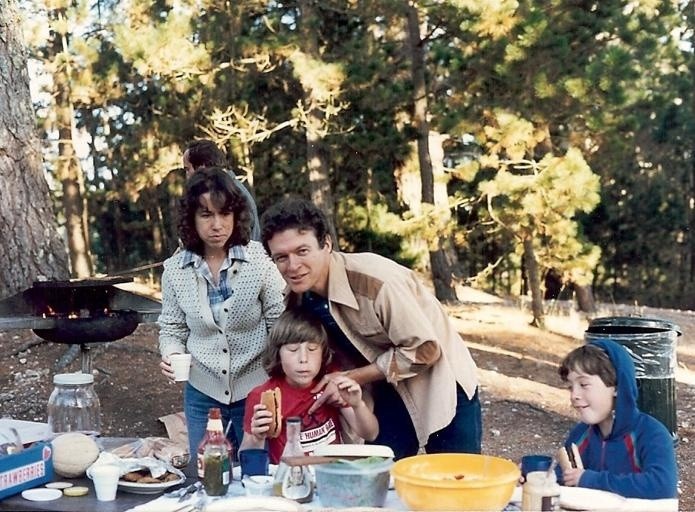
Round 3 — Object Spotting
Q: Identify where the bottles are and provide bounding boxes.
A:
[274,415,315,501]
[48,373,102,479]
[196,408,234,496]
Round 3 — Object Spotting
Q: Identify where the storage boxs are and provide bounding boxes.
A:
[1,440,56,500]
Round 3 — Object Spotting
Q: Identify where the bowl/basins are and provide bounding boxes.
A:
[388,452,521,509]
[309,454,390,507]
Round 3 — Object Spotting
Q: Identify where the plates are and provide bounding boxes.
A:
[87,457,186,494]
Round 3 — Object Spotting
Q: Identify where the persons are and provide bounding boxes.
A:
[516,338,678,500]
[155,167,288,468]
[183,139,261,467]
[259,199,484,463]
[237,307,380,465]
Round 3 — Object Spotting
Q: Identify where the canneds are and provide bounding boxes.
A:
[203,445,230,496]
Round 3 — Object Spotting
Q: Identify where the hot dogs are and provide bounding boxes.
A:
[558,442,584,472]
[260,386,282,438]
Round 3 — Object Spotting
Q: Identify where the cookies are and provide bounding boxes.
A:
[122,471,180,483]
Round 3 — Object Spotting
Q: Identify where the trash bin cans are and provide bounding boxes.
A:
[584,316,683,448]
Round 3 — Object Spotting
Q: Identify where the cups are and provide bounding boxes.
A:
[241,474,276,496]
[167,352,194,384]
[88,464,120,502]
[237,445,270,477]
